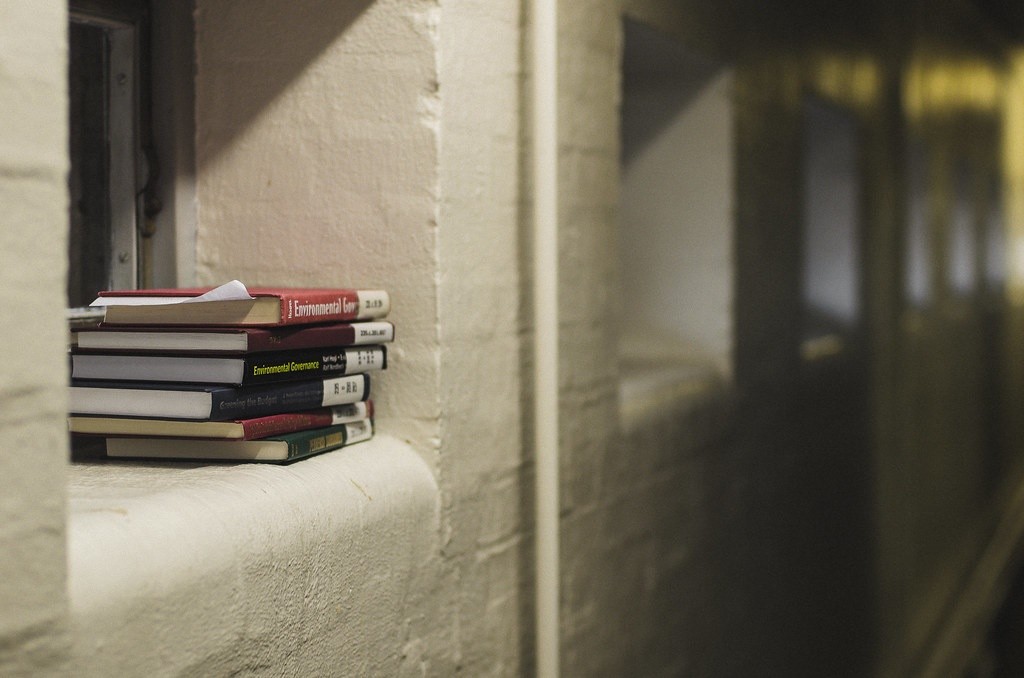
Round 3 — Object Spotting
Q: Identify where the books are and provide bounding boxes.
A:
[65,279,395,465]
